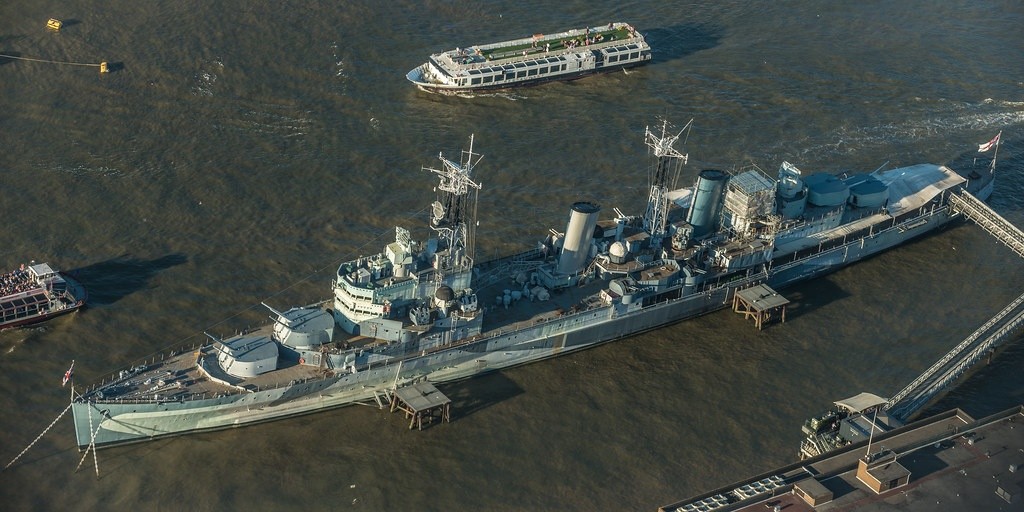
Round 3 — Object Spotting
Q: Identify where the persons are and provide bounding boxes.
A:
[532,32,601,52]
[456,46,482,64]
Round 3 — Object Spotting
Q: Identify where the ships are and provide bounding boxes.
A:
[70,113,997,454]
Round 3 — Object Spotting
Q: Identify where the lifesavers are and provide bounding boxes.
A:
[298,358,305,364]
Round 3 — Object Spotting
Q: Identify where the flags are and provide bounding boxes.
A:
[62,365,74,386]
[978,133,1000,153]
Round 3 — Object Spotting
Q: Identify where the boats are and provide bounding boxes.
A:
[406,22,652,92]
[0,261,89,330]
